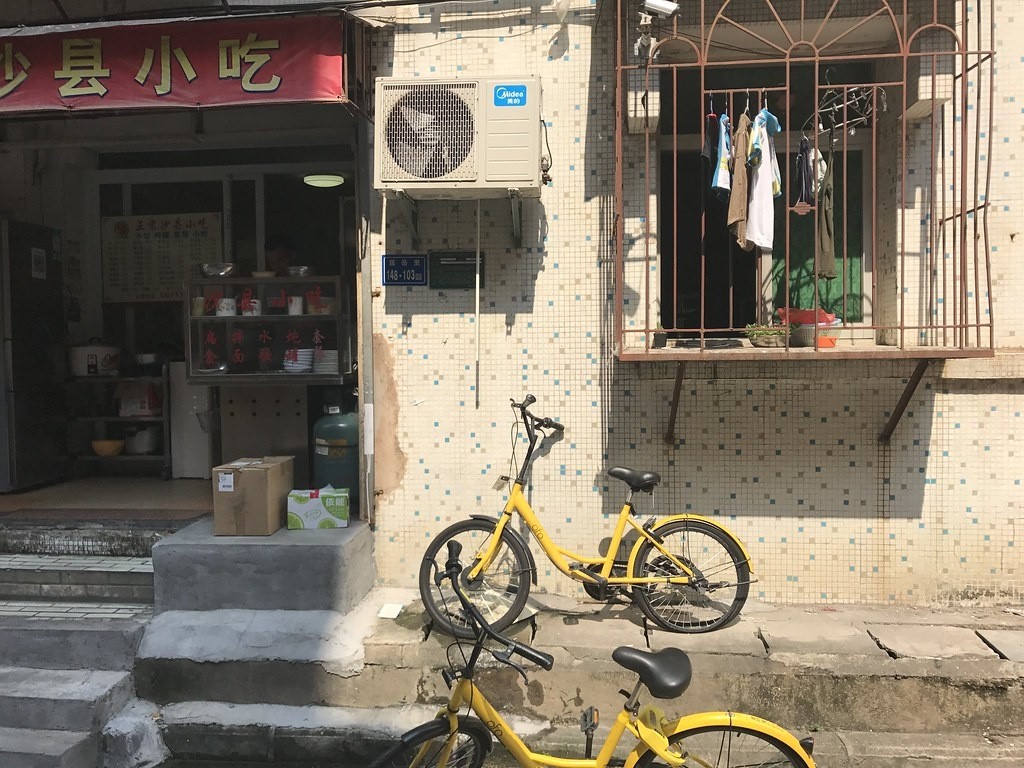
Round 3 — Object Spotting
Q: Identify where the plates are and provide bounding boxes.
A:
[200,369,229,374]
[283,349,337,372]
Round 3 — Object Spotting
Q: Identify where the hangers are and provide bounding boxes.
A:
[797,68,886,139]
[708,87,769,120]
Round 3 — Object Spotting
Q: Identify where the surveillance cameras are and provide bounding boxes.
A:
[643,0,680,18]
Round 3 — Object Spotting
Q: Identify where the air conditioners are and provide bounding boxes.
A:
[368,69,550,204]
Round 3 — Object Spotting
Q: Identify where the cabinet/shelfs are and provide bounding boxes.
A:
[62,359,172,484]
[179,265,349,393]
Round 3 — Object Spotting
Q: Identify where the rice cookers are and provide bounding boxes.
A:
[71,337,122,378]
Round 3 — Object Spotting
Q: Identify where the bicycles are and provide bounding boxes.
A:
[419,394,759,640]
[364,539,816,768]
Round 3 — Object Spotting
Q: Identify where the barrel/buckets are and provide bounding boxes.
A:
[125,423,161,455]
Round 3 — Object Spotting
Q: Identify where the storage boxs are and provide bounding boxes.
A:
[195,449,374,542]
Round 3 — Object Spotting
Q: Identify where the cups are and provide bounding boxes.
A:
[242,300,262,316]
[190,297,204,316]
[288,296,303,315]
[215,298,237,316]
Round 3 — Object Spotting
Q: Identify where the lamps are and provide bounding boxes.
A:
[297,170,349,191]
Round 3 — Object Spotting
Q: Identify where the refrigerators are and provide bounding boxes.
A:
[0,219,75,495]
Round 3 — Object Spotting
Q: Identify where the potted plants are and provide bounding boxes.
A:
[740,319,801,348]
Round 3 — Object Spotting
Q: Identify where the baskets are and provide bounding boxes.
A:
[791,318,842,347]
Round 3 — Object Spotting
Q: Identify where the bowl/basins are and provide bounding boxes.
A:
[306,296,336,315]
[92,440,125,456]
[288,265,312,277]
[251,271,277,277]
[199,263,237,279]
